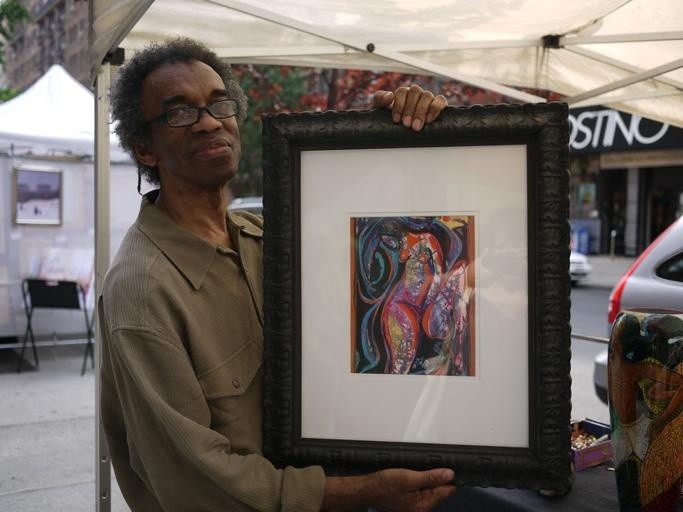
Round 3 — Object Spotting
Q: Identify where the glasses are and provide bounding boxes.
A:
[141,97,240,129]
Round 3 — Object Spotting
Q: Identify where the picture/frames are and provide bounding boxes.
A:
[260,101,572,491]
[10,164,62,227]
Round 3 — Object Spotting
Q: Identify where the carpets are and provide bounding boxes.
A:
[0,348,38,373]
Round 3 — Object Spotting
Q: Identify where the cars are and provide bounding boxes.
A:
[569,249,591,286]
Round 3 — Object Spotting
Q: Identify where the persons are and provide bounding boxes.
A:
[96,36,458,512]
[607,314,682,512]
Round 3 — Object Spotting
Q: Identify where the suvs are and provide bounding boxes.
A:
[594,213,683,406]
